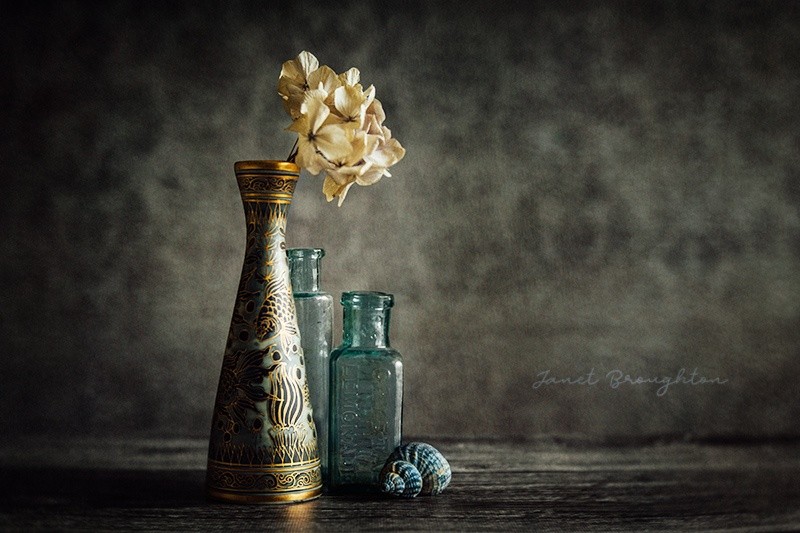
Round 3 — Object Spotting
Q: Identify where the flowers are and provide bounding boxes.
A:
[276,49,406,206]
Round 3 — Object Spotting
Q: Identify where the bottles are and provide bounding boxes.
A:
[286,247,334,482]
[329,290,404,494]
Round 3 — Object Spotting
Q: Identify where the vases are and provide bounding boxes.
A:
[206,160,323,506]
